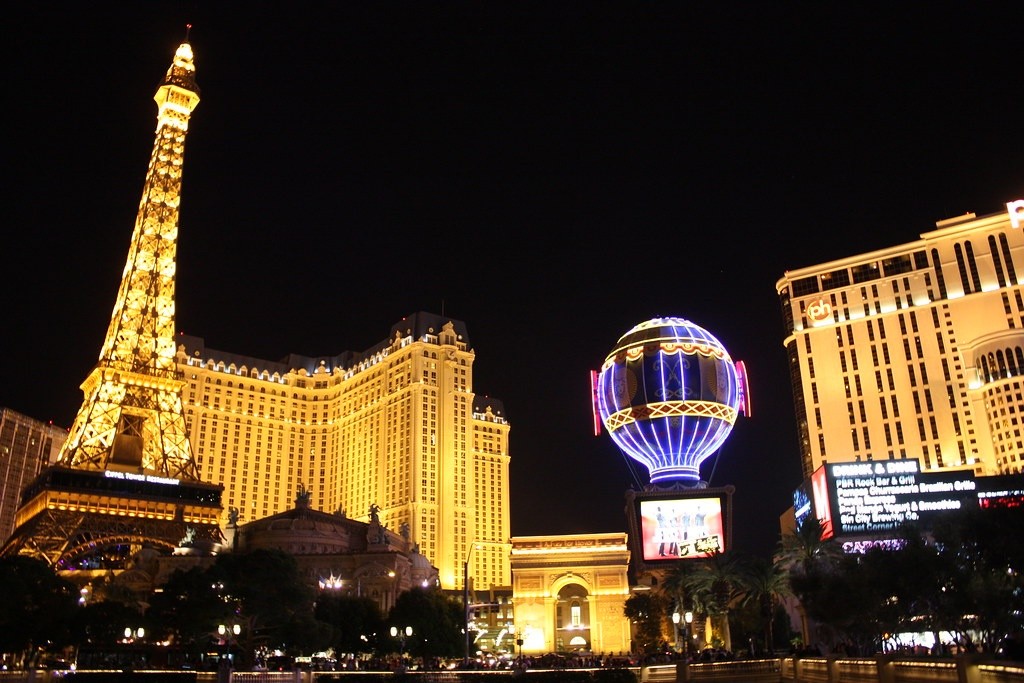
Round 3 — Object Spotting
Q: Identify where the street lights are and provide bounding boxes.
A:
[672,612,694,661]
[124,627,146,666]
[390,626,414,668]
[218,624,241,668]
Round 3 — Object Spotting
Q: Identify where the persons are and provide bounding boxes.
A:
[500,641,732,670]
[845,639,1015,665]
[653,504,709,556]
